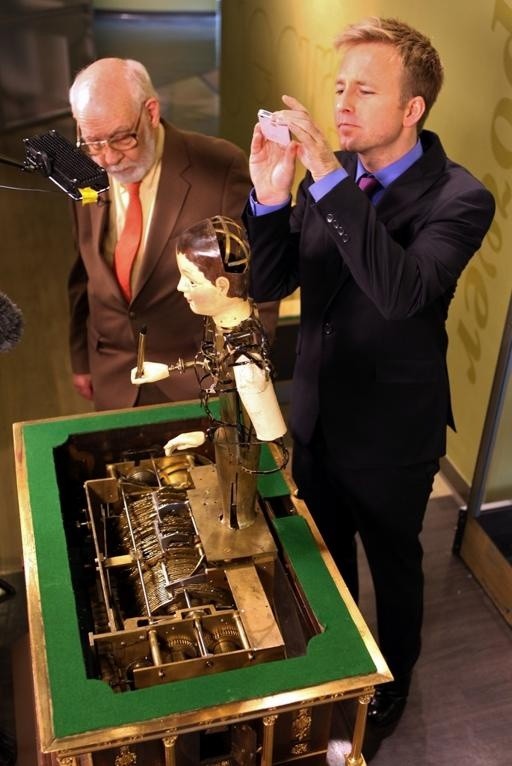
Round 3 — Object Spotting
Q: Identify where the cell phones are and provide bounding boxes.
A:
[257,109,292,146]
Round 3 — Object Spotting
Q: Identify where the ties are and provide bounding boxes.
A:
[358,177,376,193]
[114,181,142,303]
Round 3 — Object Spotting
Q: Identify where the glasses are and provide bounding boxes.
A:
[76,106,144,156]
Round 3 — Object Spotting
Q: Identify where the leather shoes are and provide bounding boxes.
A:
[367,690,405,736]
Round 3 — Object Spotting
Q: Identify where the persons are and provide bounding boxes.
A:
[66,56,280,411]
[242,18,496,728]
[127,217,287,443]
